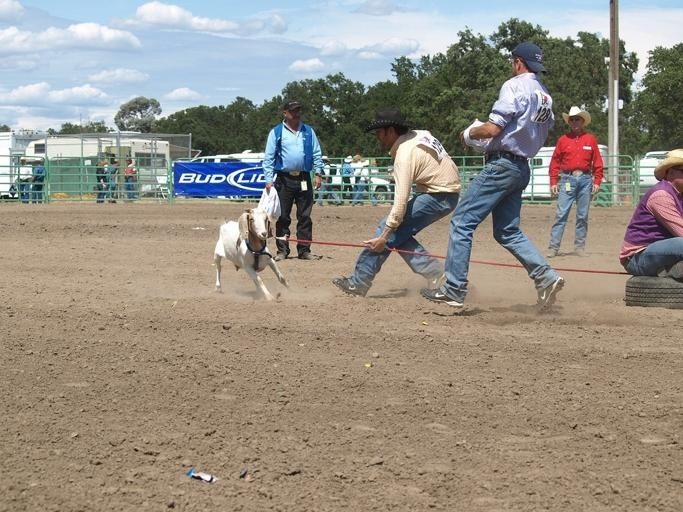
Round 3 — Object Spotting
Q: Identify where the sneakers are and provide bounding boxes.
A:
[300,252,317,260]
[575,249,587,257]
[547,250,561,258]
[657,260,683,280]
[275,253,287,260]
[537,277,564,312]
[428,273,445,289]
[332,277,368,298]
[420,289,463,307]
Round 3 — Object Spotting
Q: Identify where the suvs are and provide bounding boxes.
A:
[631,148,674,187]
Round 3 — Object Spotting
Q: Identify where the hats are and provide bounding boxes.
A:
[284,102,302,111]
[512,42,548,73]
[343,155,369,166]
[321,155,329,161]
[363,108,413,134]
[654,148,683,181]
[562,105,591,128]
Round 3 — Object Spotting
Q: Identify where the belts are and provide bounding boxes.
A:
[283,170,304,176]
[623,257,632,269]
[491,151,527,160]
[563,169,587,176]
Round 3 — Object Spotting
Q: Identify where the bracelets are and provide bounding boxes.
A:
[315,173,321,177]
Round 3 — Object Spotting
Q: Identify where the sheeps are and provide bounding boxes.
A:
[212,207,292,302]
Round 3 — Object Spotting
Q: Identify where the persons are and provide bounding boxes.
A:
[93,149,138,205]
[31,158,47,204]
[315,154,342,207]
[261,102,327,261]
[333,107,463,298]
[418,42,564,315]
[17,158,32,203]
[617,148,682,279]
[546,106,603,258]
[339,154,395,207]
